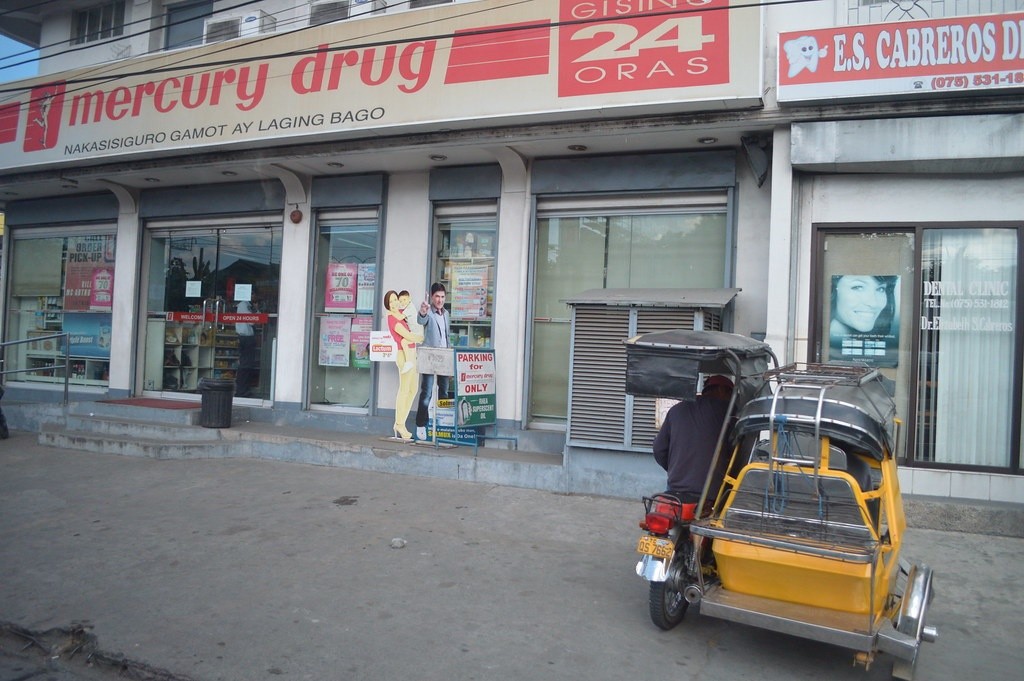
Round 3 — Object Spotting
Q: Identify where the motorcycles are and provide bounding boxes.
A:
[620,330,939,680]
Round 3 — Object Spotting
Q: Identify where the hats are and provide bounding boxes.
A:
[701,374,735,394]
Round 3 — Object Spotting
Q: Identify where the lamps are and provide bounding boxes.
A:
[740,135,773,188]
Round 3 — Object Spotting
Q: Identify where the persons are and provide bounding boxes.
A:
[384,290,423,439]
[387,290,424,374]
[235,288,258,398]
[416,283,451,439]
[831,275,896,338]
[653,376,743,573]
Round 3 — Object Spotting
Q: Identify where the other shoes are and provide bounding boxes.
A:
[402,363,413,373]
[417,426,426,440]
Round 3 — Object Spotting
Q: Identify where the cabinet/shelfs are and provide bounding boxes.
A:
[437,223,497,392]
[17,250,112,386]
[144,317,262,391]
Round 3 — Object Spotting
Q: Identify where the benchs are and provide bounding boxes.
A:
[745,444,877,534]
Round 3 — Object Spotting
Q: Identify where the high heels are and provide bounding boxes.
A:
[393,423,412,440]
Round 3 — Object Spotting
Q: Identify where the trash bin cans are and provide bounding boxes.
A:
[200,377,235,429]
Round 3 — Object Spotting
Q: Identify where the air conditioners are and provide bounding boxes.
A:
[202,8,276,45]
[308,0,388,27]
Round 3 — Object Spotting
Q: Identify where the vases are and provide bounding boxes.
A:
[200,377,234,428]
